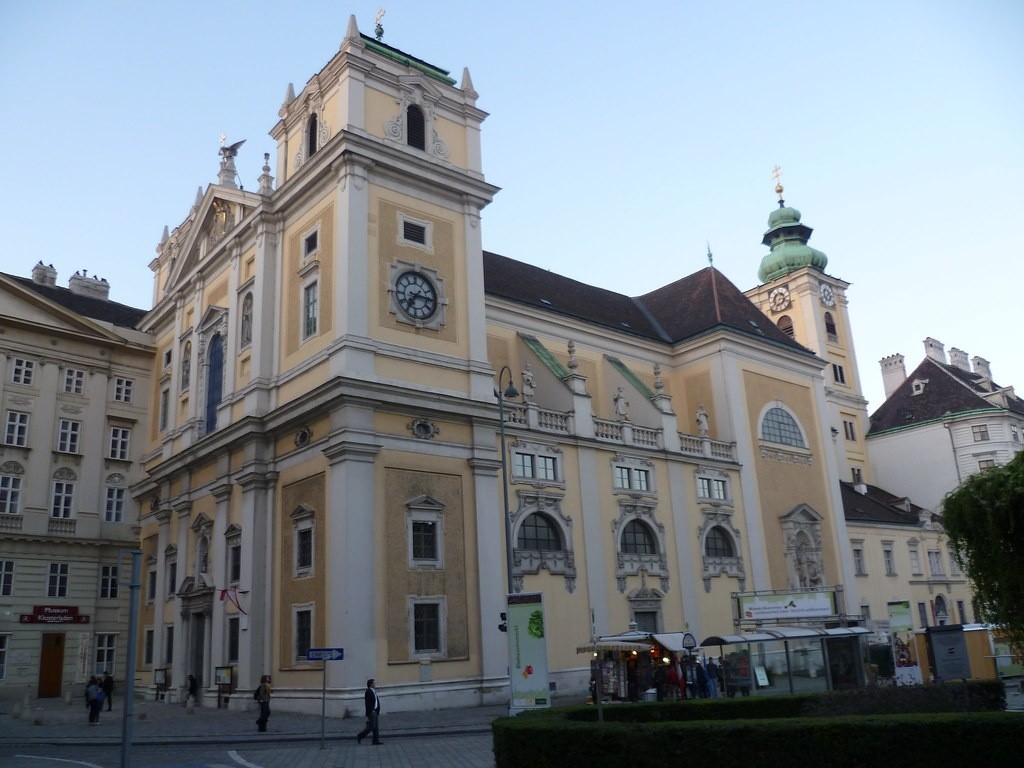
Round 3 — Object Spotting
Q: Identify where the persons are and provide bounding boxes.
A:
[357,678,385,745]
[614,387,631,421]
[182,675,198,707]
[255,675,272,732]
[84,670,114,725]
[521,363,535,404]
[695,404,709,436]
[589,651,751,704]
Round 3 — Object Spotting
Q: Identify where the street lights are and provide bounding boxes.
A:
[498,366,519,595]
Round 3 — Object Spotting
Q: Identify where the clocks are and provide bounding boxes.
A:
[820,284,835,307]
[392,269,444,324]
[769,287,791,313]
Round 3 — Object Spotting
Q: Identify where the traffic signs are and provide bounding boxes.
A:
[308,648,344,748]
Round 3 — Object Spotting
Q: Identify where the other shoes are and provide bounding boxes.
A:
[373,741,384,745]
[357,734,361,745]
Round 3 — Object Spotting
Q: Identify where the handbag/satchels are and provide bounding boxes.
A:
[95,686,104,702]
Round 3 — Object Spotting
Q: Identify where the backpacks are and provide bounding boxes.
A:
[254,685,261,700]
